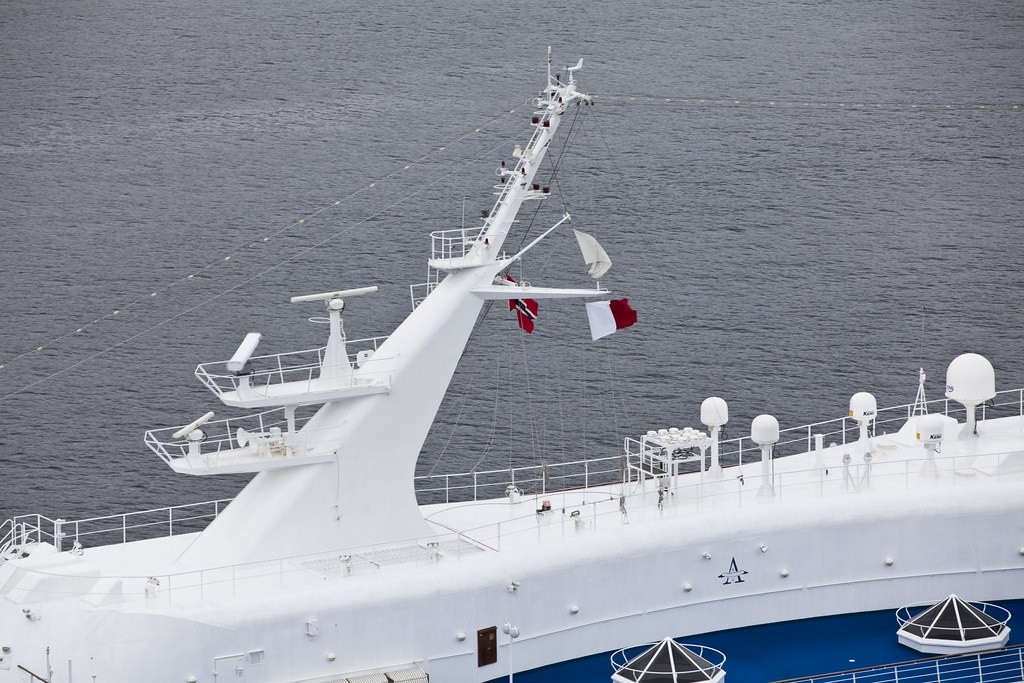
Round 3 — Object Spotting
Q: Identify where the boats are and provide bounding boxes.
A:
[0,46,1024,683]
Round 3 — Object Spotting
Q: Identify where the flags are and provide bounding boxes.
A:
[586,298,637,340]
[573,229,612,279]
[507,275,538,334]
